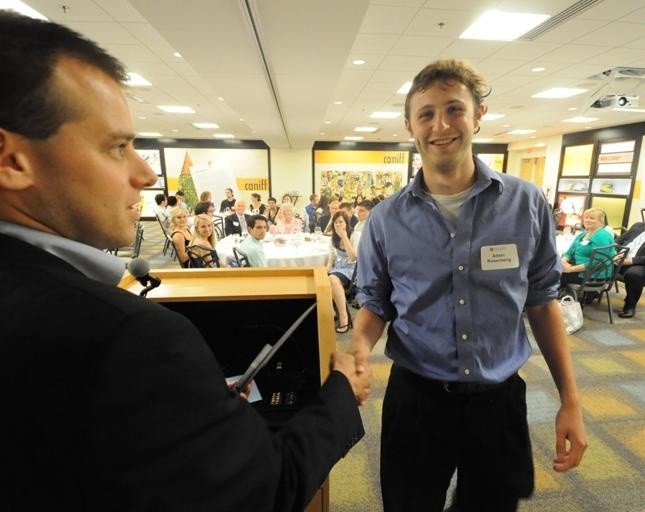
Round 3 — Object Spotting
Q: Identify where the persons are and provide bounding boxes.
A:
[560,209,613,286]
[339,203,357,237]
[190,202,214,235]
[153,191,184,212]
[200,190,212,202]
[0,9,371,512]
[321,200,340,236]
[269,203,302,235]
[250,193,266,215]
[339,195,384,204]
[349,200,373,242]
[236,215,268,267]
[188,214,216,267]
[326,210,357,333]
[219,188,236,212]
[348,57,589,512]
[224,199,251,236]
[267,197,280,225]
[282,194,292,203]
[170,206,192,268]
[305,194,326,234]
[614,222,645,318]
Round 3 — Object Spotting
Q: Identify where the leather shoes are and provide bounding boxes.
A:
[618,308,635,318]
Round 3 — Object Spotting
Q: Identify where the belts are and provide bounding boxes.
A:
[415,381,509,394]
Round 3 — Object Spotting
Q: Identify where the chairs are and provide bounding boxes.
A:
[232,247,250,267]
[111,231,144,259]
[185,245,220,268]
[613,227,628,241]
[166,234,187,269]
[561,244,630,324]
[213,214,223,239]
[155,213,169,256]
[344,262,358,329]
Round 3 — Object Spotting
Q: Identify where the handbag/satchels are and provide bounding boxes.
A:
[558,294,584,335]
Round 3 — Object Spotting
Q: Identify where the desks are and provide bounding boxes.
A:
[117,265,335,512]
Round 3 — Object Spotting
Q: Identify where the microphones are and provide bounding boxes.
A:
[128,258,161,298]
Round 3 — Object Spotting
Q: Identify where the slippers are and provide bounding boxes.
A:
[336,319,349,334]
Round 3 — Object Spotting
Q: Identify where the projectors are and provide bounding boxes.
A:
[600,92,640,111]
